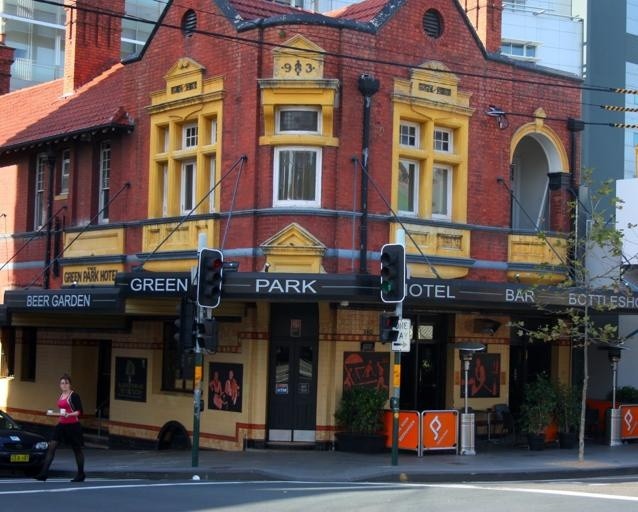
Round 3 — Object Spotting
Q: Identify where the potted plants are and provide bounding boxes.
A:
[333,382,389,453]
[518,369,583,450]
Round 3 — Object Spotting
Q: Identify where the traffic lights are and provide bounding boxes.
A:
[198,317,219,354]
[377,240,406,304]
[376,311,400,346]
[194,246,225,309]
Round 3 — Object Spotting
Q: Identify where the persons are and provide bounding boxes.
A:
[210,369,239,410]
[363,359,388,391]
[34,373,85,482]
[472,357,487,396]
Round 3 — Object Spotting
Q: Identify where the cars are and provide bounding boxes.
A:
[0,409,50,479]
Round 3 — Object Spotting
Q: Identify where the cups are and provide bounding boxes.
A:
[60,409,66,415]
[47,409,53,414]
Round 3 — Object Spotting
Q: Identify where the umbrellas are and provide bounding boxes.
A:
[345,353,363,364]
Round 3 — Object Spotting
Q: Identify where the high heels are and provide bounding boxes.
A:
[70,475,86,481]
[33,474,47,481]
